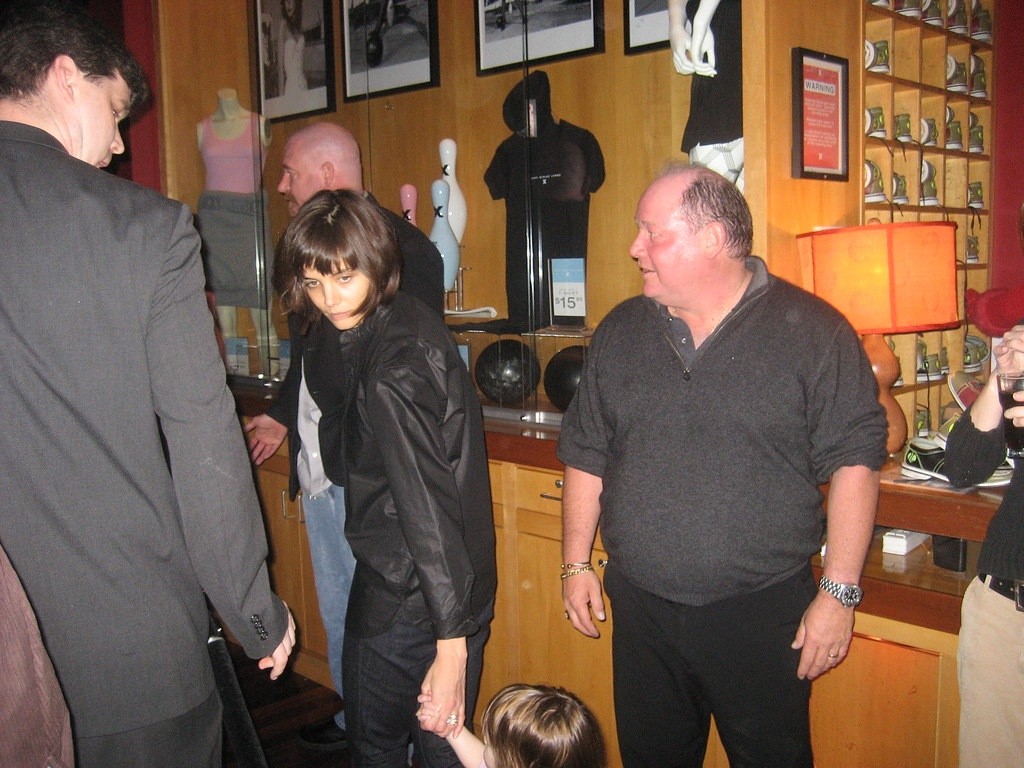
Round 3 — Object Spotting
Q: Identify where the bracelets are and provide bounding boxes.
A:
[561,556,593,579]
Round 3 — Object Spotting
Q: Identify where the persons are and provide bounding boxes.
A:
[196,88,281,377]
[667,0,743,194]
[559,162,888,767]
[0,0,297,768]
[944,318,1024,768]
[277,0,308,96]
[276,191,496,768]
[243,122,445,768]
[417,683,606,768]
[0,544,75,768]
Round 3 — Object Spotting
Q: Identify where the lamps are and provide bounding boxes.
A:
[795,221,960,453]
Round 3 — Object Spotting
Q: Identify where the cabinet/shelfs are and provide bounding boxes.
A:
[848,0,997,448]
[210,413,942,768]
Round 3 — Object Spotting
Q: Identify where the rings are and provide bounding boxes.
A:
[447,714,459,725]
[565,613,570,620]
[828,654,838,658]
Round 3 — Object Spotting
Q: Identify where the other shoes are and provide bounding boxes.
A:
[295,717,348,750]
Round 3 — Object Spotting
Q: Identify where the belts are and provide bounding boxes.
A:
[977,571,1024,612]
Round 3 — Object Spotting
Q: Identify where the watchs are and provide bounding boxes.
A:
[819,575,864,606]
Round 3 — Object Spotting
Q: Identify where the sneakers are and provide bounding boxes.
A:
[948,371,987,410]
[883,335,987,388]
[901,401,1014,488]
[860,0,992,266]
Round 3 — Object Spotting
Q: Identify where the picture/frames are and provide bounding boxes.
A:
[473,0,607,77]
[253,0,337,124]
[622,0,692,57]
[339,0,440,104]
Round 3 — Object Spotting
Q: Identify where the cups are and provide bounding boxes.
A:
[997,372,1024,458]
